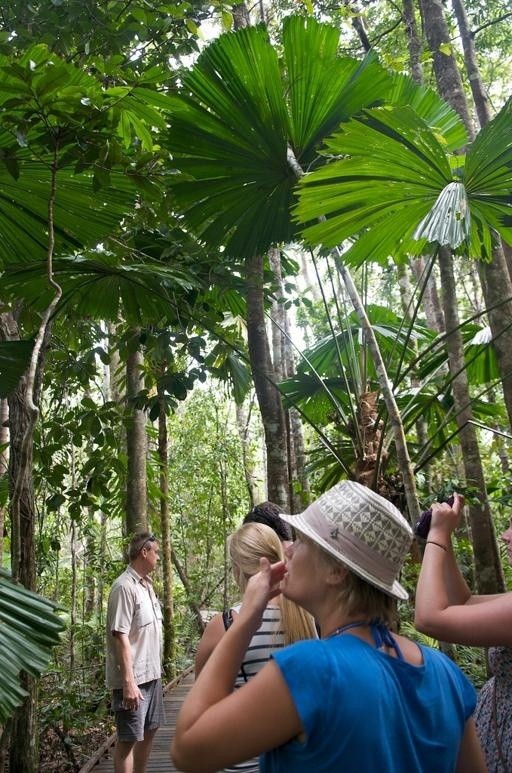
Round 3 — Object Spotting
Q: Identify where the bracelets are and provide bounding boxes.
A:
[425,541,447,551]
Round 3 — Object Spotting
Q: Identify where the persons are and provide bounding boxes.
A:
[171,480,487,773]
[243,502,293,553]
[414,492,512,773]
[195,522,319,773]
[107,532,168,772]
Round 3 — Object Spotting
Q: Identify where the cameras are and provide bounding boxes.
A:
[446,494,455,508]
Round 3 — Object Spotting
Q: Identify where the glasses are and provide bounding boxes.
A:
[251,506,273,523]
[139,535,155,550]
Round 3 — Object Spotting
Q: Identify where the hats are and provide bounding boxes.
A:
[278,477,416,603]
